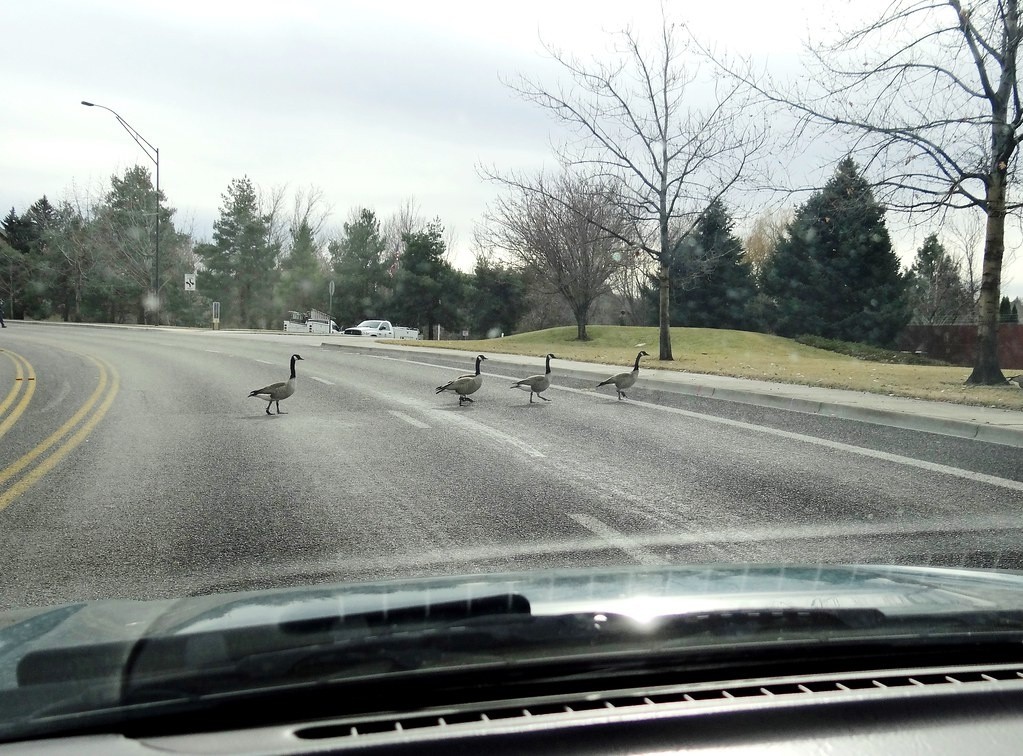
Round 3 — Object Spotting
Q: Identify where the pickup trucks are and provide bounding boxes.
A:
[344,320,419,341]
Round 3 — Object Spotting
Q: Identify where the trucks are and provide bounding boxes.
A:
[284,309,342,334]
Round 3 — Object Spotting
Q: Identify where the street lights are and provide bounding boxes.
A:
[81,101,159,328]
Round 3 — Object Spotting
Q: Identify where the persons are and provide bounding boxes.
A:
[0,299,7,328]
[619,310,626,326]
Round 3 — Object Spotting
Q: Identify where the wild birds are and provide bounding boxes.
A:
[247,353,305,416]
[509,353,557,404]
[435,355,489,406]
[595,350,651,400]
